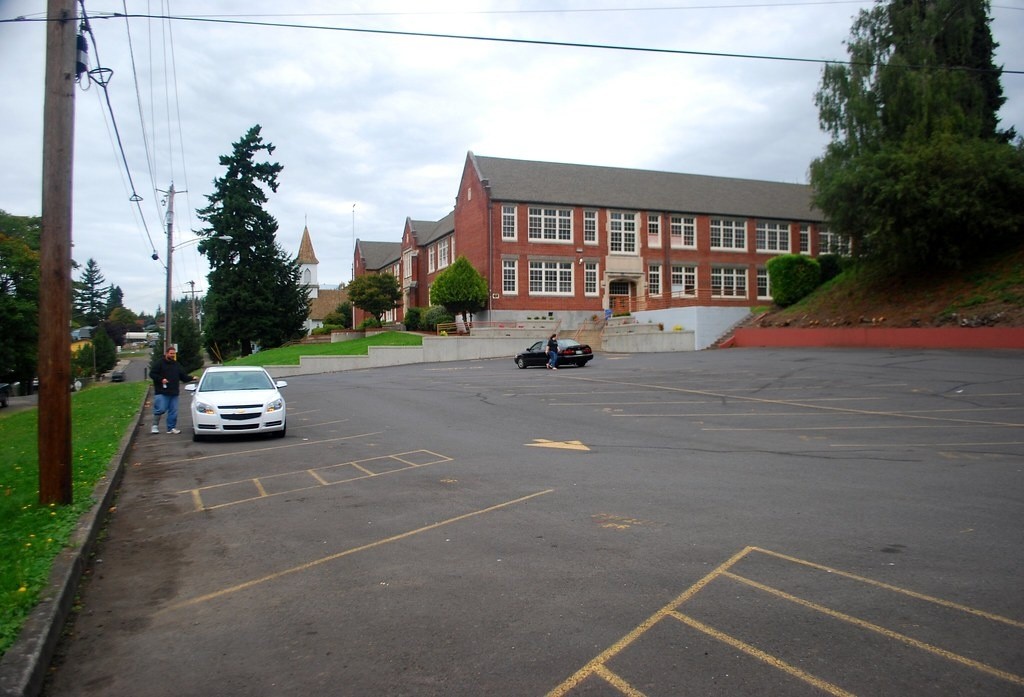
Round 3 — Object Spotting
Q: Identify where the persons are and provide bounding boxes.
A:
[545,335,560,370]
[603,308,612,320]
[148,347,201,435]
[439,328,448,337]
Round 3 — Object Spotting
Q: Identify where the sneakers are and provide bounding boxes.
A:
[167,428,182,434]
[150,424,159,433]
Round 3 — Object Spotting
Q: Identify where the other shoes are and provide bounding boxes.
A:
[546,363,558,370]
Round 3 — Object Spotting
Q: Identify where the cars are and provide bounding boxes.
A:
[512,338,593,369]
[0,382,11,409]
[184,365,288,440]
[149,341,155,347]
[33,378,40,388]
[113,371,126,381]
